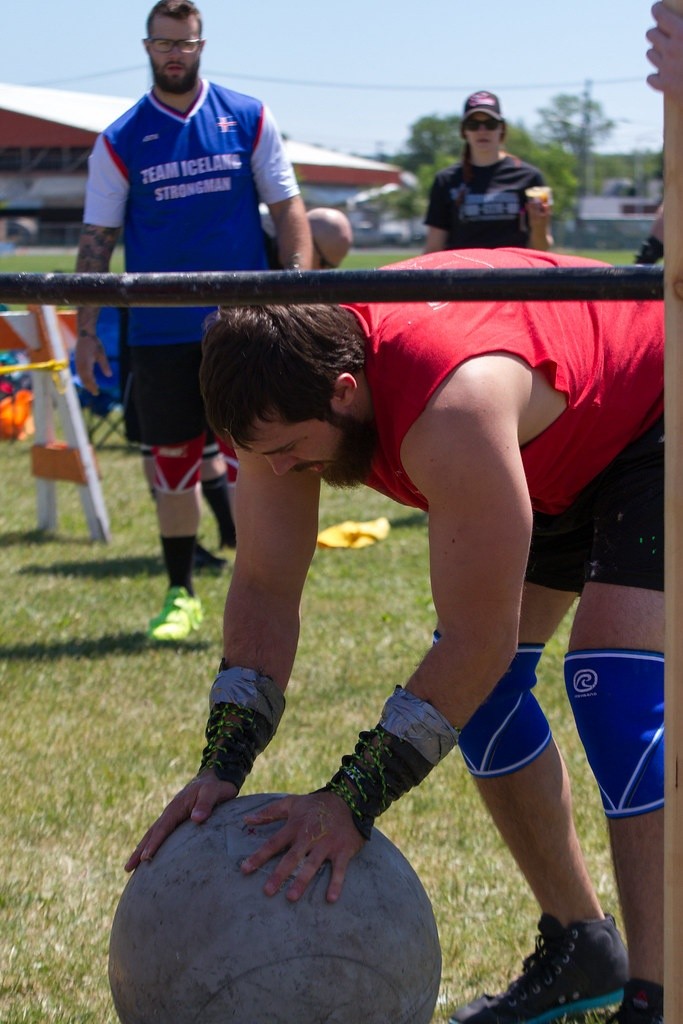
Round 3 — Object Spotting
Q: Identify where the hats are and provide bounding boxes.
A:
[464,91,503,122]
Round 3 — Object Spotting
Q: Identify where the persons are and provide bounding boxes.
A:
[140,201,354,572]
[76,0,315,641]
[634,1,682,265]
[422,90,554,257]
[123,247,666,1024]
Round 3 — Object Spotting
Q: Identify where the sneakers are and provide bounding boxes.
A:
[605,979,665,1024]
[447,912,628,1024]
[147,586,203,640]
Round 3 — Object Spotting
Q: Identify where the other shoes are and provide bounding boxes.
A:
[189,533,237,573]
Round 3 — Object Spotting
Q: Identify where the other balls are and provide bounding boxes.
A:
[109,792,442,1024]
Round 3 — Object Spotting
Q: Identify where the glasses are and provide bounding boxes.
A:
[148,38,201,54]
[466,118,504,131]
[313,240,337,270]
